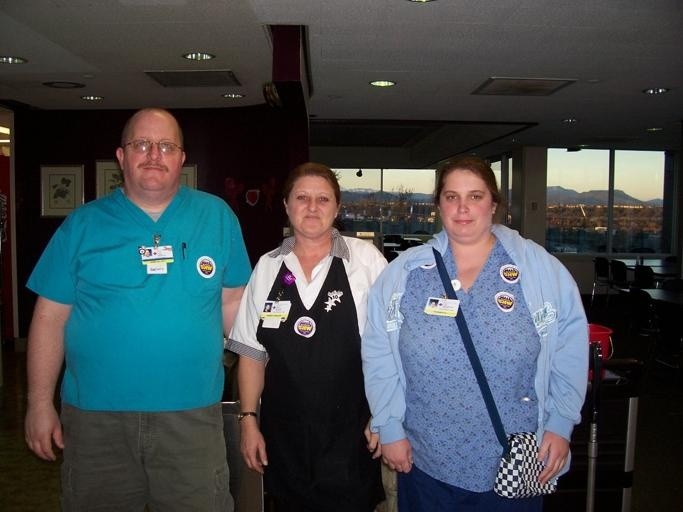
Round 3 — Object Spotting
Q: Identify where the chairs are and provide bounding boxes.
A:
[590,255,668,367]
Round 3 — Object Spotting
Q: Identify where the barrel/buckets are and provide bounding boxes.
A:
[587,323,615,379]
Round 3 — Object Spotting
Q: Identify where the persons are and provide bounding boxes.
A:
[359,154,592,511]
[22,106,255,511]
[226,161,391,512]
[143,249,152,259]
[263,303,270,312]
[429,299,438,309]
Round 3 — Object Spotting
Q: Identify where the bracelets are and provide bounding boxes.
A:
[236,411,259,423]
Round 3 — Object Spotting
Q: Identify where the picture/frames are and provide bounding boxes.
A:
[178,162,199,189]
[38,159,118,219]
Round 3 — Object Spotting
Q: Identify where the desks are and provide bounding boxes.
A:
[607,258,683,306]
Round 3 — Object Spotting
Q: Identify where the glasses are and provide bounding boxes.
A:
[121,137,183,155]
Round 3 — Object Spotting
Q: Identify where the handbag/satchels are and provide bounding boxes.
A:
[493,430,561,500]
